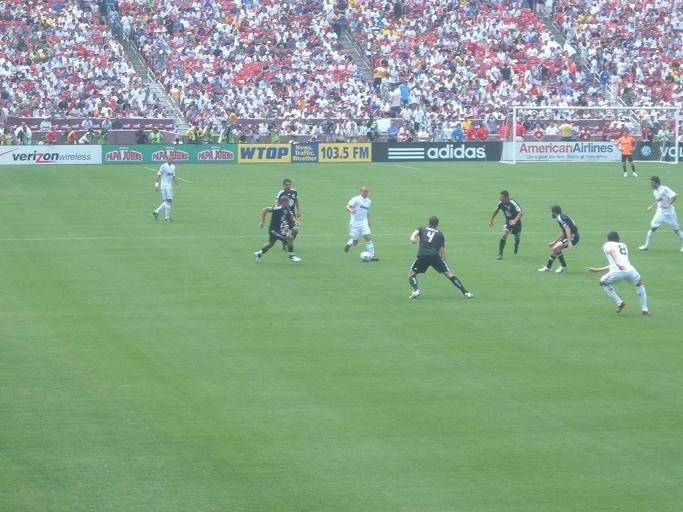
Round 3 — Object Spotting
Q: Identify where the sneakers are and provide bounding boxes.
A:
[345,243,350,251]
[255,250,262,262]
[538,265,567,273]
[289,255,300,262]
[409,289,420,299]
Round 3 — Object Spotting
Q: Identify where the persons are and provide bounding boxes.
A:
[538,205,580,273]
[409,216,474,299]
[153,155,178,221]
[254,196,302,262]
[616,131,639,178]
[276,179,300,250]
[586,231,650,315]
[488,190,523,259]
[637,176,683,252]
[346,187,379,261]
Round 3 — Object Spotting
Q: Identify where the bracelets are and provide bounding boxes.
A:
[155,182,159,187]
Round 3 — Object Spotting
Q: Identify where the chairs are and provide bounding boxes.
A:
[0,0,683,144]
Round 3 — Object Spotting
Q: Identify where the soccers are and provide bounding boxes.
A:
[361,252,371,262]
